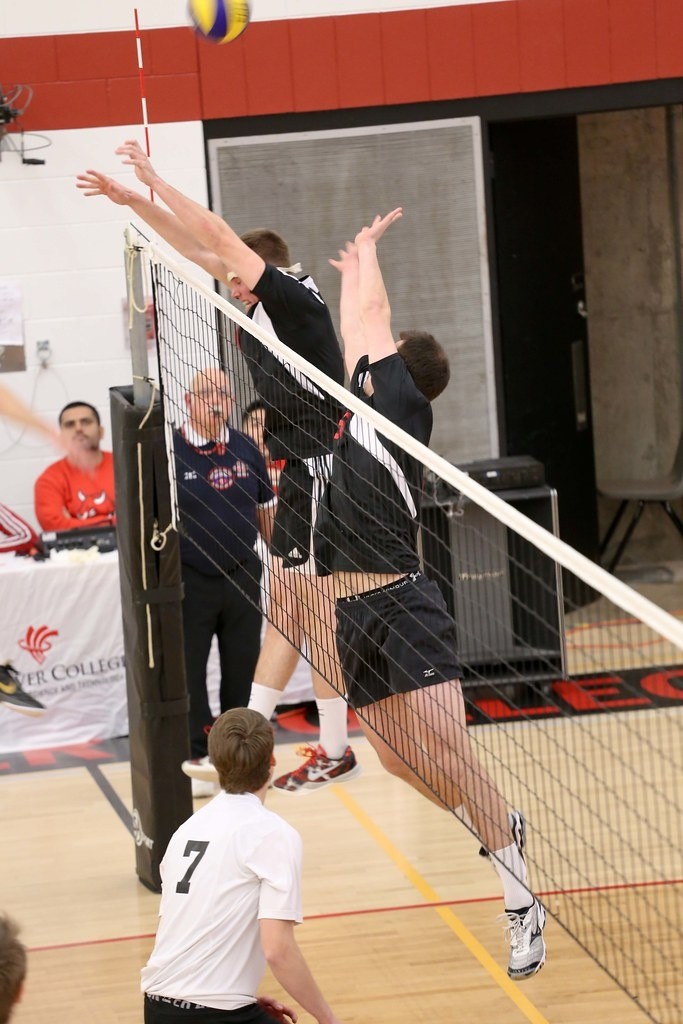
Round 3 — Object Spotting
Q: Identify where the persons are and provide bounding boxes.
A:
[240,399,287,496]
[313,207,548,983]
[34,401,117,533]
[168,365,278,795]
[75,138,362,797]
[0,500,38,555]
[140,705,338,1024]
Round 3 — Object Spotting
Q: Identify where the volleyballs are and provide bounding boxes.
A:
[183,0,252,43]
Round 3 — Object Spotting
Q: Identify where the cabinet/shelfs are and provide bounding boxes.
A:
[416,484,568,688]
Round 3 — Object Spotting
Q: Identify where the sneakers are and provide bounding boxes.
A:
[2,663,51,715]
[182,744,361,795]
[478,808,549,978]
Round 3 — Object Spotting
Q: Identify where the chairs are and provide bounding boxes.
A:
[592,424,683,575]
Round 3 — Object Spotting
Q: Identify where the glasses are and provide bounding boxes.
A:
[189,389,232,401]
